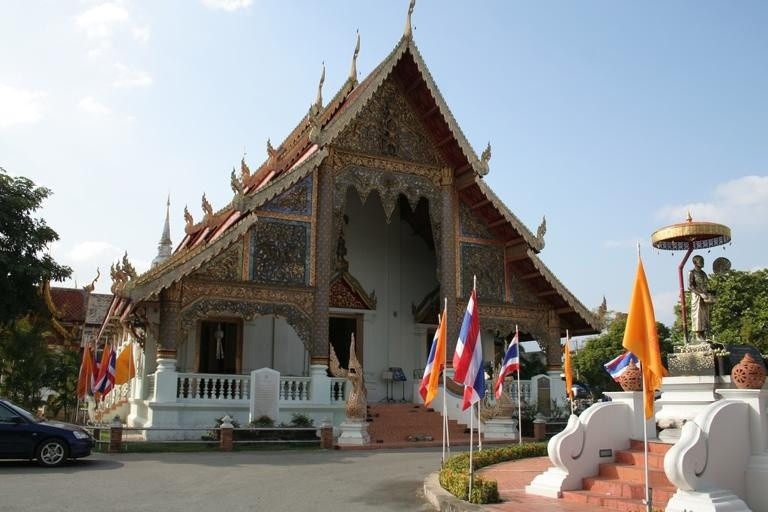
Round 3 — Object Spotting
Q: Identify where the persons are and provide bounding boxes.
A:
[687,255,715,343]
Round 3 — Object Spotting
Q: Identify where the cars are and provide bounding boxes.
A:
[0,396,96,467]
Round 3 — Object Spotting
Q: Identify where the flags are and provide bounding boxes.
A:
[564,341,572,399]
[494,331,521,400]
[621,254,663,419]
[602,350,640,384]
[418,287,486,413]
[76,340,135,402]
